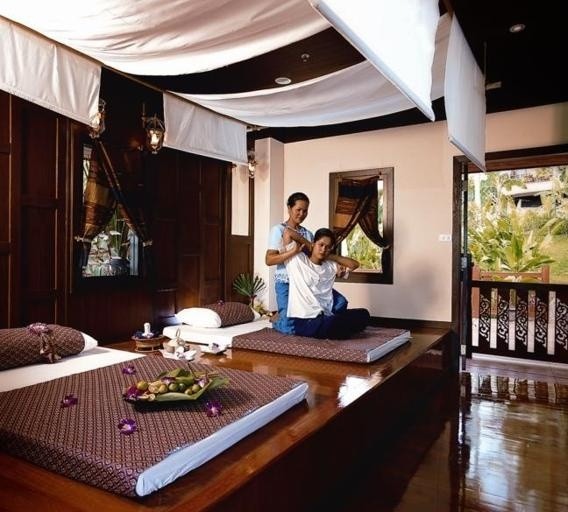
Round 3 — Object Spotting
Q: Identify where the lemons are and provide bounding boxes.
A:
[136,381,200,395]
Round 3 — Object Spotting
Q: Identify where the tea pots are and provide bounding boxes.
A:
[164,328,185,352]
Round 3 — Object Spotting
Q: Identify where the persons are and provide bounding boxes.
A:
[277,225,371,340]
[265,191,349,337]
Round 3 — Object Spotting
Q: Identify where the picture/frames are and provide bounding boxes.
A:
[80,134,132,281]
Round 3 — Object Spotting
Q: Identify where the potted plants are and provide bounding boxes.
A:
[173,299,263,330]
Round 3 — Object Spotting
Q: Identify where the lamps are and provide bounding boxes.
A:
[246,146,259,181]
[86,95,108,146]
[140,100,167,158]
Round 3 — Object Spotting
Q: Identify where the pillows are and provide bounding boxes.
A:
[0,322,100,373]
